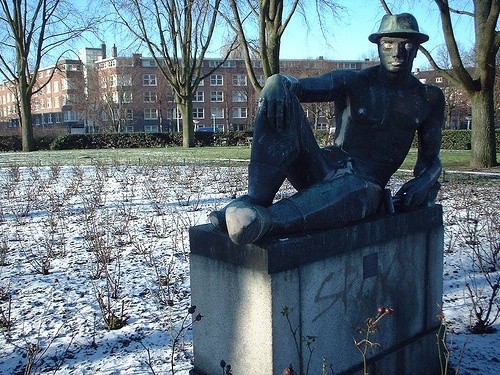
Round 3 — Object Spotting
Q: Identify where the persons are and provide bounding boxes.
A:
[209,11,446,245]
